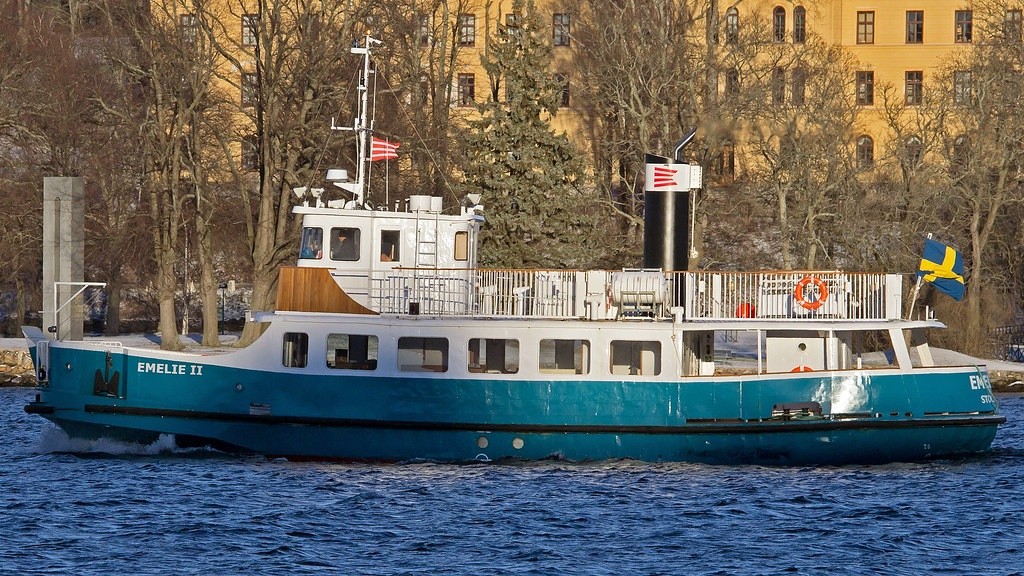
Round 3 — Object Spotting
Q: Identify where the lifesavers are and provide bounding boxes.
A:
[791,366,813,372]
[795,277,828,310]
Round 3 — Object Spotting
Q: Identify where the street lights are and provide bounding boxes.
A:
[219,283,228,334]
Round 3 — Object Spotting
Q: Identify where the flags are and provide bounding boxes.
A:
[917,239,967,300]
[371,137,400,162]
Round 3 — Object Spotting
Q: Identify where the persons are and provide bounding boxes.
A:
[332,230,356,258]
[380,240,393,261]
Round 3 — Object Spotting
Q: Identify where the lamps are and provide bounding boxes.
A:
[293,186,310,207]
[311,188,325,208]
[467,192,482,206]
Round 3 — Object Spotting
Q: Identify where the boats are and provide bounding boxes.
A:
[20,29,1007,468]
